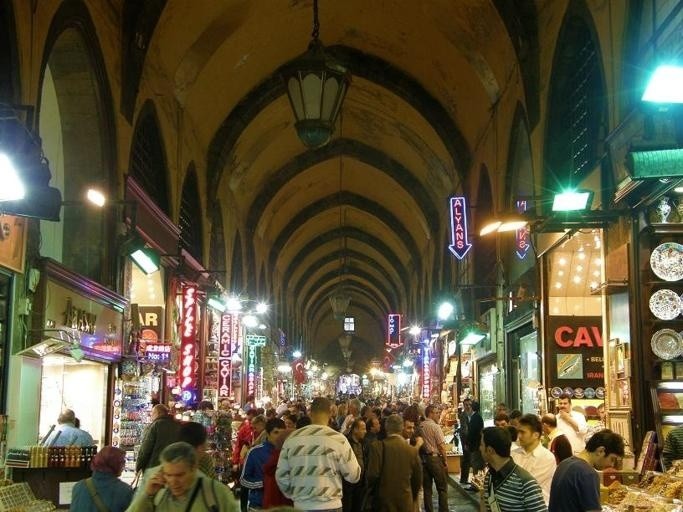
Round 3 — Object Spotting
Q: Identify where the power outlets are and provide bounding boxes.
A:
[16,297,30,315]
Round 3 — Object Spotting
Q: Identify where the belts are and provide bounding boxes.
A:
[429,453,438,456]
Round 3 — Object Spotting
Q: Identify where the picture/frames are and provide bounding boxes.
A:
[607,336,633,455]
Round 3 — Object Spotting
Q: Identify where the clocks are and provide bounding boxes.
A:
[120,358,136,375]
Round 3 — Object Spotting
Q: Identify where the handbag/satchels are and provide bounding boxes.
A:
[360,487,380,512]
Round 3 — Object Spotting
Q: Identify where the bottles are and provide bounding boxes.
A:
[6,448,30,461]
[30,444,97,469]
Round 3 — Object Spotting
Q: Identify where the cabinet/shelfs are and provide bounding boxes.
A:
[110,379,146,450]
[549,396,605,427]
[642,379,683,474]
[635,223,682,383]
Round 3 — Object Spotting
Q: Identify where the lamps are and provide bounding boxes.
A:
[328,109,354,375]
[621,106,683,182]
[195,287,230,314]
[454,293,541,349]
[478,213,540,236]
[278,0,353,149]
[0,101,62,221]
[128,247,187,282]
[550,188,609,221]
[20,323,86,362]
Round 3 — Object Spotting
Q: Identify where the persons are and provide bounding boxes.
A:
[44,389,683,512]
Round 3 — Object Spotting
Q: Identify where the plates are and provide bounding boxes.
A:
[550,386,562,399]
[583,386,594,399]
[573,387,584,399]
[594,387,604,399]
[650,328,682,360]
[647,241,682,282]
[648,288,680,323]
[562,386,573,398]
